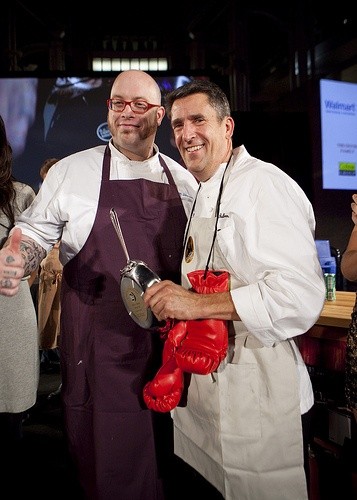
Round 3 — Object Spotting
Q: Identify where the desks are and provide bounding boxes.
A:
[296,290,357,500]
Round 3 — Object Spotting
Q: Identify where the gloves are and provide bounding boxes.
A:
[143,339,184,412]
[167,270,229,375]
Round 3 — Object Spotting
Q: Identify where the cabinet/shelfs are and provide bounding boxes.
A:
[228,0,357,114]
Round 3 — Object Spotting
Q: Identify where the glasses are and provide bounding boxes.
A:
[106,99,160,114]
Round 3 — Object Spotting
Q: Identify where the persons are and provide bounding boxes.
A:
[0,71,203,500]
[339,193,357,500]
[28,158,63,373]
[0,115,44,500]
[143,79,326,500]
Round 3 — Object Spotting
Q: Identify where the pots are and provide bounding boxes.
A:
[110,207,174,334]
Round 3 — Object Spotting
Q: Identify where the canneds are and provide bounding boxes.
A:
[323,273,336,301]
